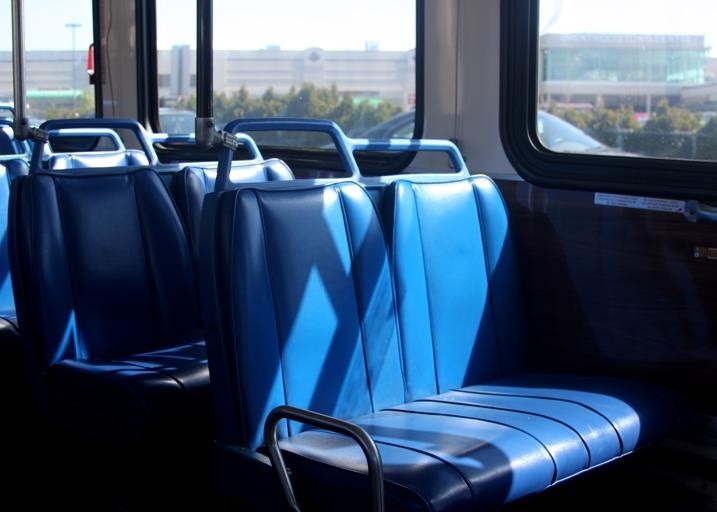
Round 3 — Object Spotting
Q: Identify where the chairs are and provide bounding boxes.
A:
[0,105,685,512]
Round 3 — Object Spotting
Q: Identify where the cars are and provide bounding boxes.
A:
[321,103,645,159]
[157,106,196,134]
